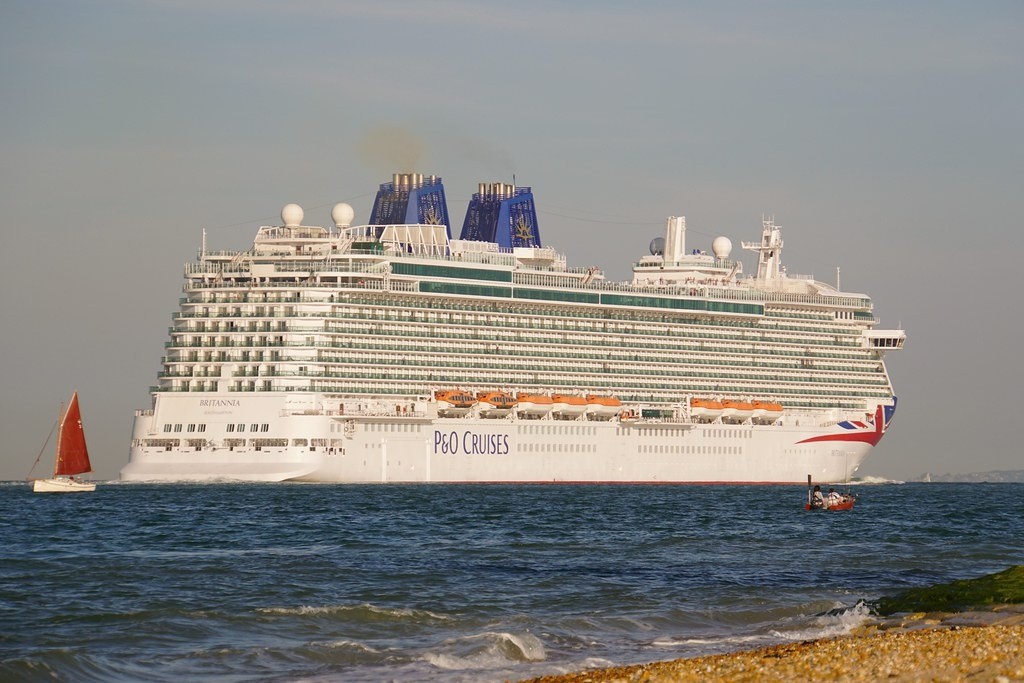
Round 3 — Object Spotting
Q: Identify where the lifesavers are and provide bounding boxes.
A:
[622,410,631,419]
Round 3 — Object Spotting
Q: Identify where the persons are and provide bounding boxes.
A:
[811,486,852,510]
[69,475,74,481]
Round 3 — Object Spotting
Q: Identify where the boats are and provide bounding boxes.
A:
[690,397,725,423]
[805,473,857,511]
[474,392,518,419]
[435,390,477,418]
[551,393,589,420]
[751,400,785,424]
[515,391,554,420]
[721,399,754,423]
[586,395,623,421]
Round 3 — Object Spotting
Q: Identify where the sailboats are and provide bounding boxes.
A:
[25,390,97,493]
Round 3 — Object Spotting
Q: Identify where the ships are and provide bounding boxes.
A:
[113,168,910,488]
